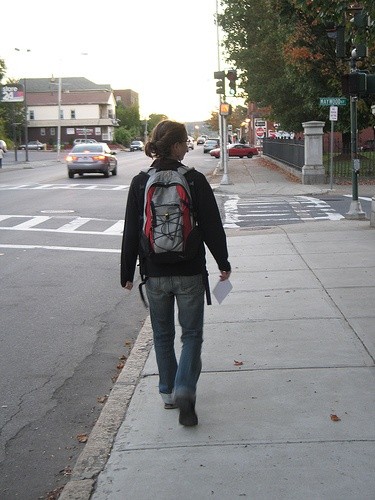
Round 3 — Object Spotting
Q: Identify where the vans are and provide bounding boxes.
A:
[72,139,97,148]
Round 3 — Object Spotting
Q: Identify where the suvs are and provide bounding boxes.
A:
[201,135,208,141]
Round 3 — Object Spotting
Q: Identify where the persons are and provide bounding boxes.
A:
[0,147,4,168]
[240,138,245,144]
[121,120,231,427]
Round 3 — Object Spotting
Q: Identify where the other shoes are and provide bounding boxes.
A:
[175,396,198,427]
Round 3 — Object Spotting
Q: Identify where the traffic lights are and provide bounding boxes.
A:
[214,71,225,96]
[220,103,232,116]
[326,26,344,59]
[340,72,362,94]
[227,71,237,90]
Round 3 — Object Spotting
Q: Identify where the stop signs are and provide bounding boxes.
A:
[256,128,264,137]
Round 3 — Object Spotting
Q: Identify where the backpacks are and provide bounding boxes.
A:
[142,164,198,257]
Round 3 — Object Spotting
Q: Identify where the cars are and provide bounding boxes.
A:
[18,141,47,150]
[203,140,218,153]
[197,137,205,145]
[186,137,195,150]
[267,130,291,139]
[129,140,144,152]
[209,143,259,158]
[66,142,117,178]
